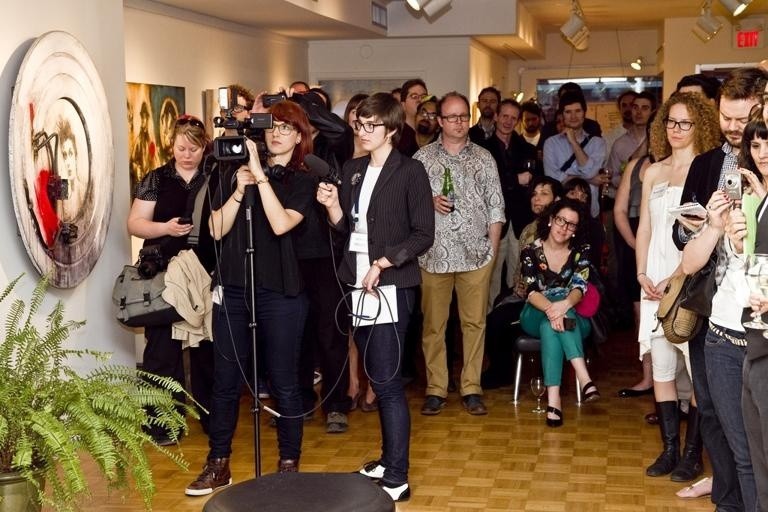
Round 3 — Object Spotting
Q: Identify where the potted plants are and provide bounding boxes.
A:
[0,265,210,512]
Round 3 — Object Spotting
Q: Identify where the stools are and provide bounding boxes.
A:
[509,335,589,406]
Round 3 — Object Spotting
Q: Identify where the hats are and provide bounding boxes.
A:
[140,102,152,119]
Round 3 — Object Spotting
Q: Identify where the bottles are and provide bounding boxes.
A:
[442,167,456,213]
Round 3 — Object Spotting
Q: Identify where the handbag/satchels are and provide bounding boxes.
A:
[655,234,716,344]
[112,163,218,327]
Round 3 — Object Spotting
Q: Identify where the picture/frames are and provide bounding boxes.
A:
[122,81,186,203]
[308,71,428,119]
[7,29,116,289]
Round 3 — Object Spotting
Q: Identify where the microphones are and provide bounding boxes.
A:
[302,153,344,186]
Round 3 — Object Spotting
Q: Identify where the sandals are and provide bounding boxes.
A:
[362,390,376,413]
[346,388,358,409]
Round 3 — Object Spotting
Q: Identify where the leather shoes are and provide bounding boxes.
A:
[615,385,658,396]
[463,394,488,417]
[420,394,447,414]
[647,410,687,423]
[545,400,566,428]
[156,433,176,448]
[580,380,600,405]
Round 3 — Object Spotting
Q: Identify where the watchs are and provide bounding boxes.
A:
[255,175,269,186]
[373,259,384,274]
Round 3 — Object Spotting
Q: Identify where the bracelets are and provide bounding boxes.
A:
[229,193,242,205]
[633,271,648,279]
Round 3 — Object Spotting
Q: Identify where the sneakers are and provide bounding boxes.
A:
[312,370,319,383]
[275,458,301,477]
[372,478,411,503]
[255,382,270,400]
[268,408,315,426]
[325,411,348,433]
[185,456,232,496]
[351,462,384,482]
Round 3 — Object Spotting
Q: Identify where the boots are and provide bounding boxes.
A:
[644,397,682,477]
[670,404,705,482]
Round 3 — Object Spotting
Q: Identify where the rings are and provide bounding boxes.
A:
[707,206,715,212]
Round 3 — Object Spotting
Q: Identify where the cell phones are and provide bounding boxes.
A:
[564,317,576,331]
[178,213,192,224]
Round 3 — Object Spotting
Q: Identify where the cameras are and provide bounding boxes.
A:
[722,168,744,201]
[262,91,287,108]
[137,245,168,280]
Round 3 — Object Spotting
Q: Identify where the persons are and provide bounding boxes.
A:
[343,94,378,411]
[541,83,602,141]
[203,85,251,135]
[667,68,766,504]
[519,198,600,429]
[317,92,434,501]
[521,102,556,149]
[484,98,540,320]
[185,102,318,498]
[604,91,638,170]
[634,92,722,484]
[127,118,219,446]
[741,80,768,512]
[130,102,154,184]
[395,78,429,136]
[400,96,456,395]
[543,92,613,218]
[563,178,622,350]
[249,88,354,433]
[160,112,176,147]
[556,111,568,134]
[705,120,768,512]
[410,92,506,416]
[486,177,567,330]
[31,118,86,219]
[644,75,722,423]
[613,109,672,399]
[467,85,502,144]
[288,81,311,94]
[607,90,657,327]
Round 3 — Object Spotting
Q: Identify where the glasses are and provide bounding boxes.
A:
[232,102,252,114]
[661,116,694,132]
[417,108,438,121]
[405,92,428,100]
[551,213,579,233]
[264,121,301,137]
[352,119,387,134]
[440,114,472,123]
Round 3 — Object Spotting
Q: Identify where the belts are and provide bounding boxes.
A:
[705,322,748,348]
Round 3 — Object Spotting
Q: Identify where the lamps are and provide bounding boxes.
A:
[511,91,525,103]
[406,1,428,12]
[423,0,453,18]
[566,26,591,46]
[576,38,589,50]
[696,13,724,37]
[631,56,643,71]
[690,23,712,43]
[560,13,586,39]
[720,0,747,17]
